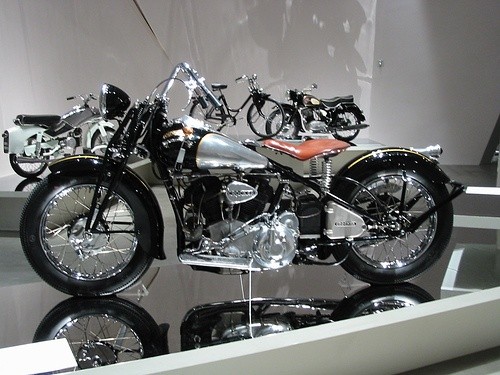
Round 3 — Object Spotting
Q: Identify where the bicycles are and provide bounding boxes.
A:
[189,74,284,138]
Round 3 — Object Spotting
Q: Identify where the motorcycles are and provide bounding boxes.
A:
[31,283,437,375]
[266,83,370,142]
[1,82,131,178]
[19,62,467,299]
